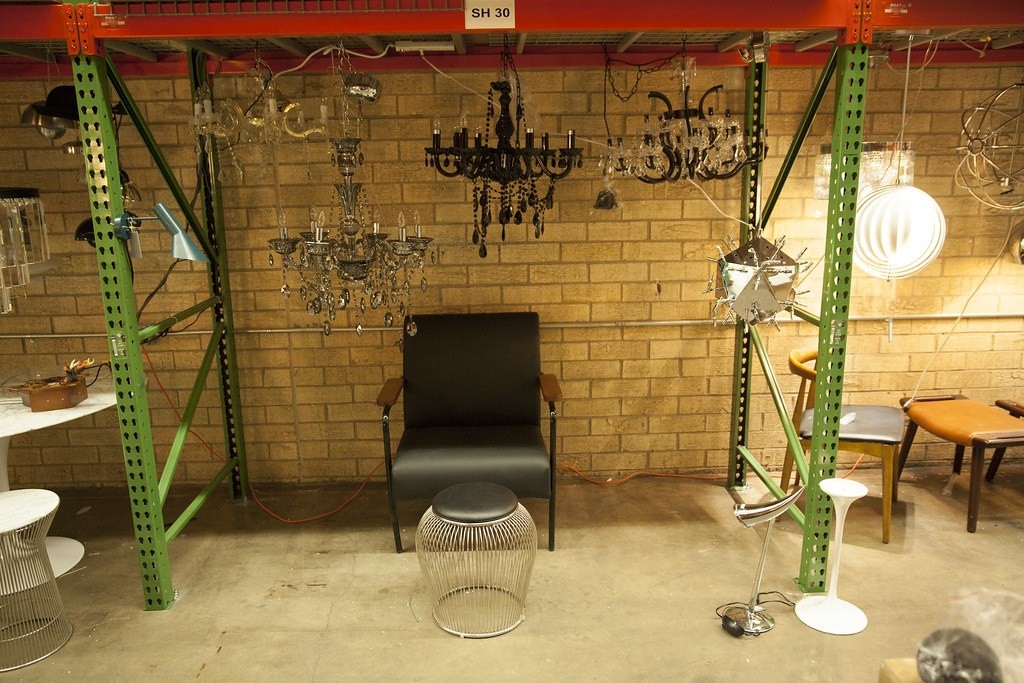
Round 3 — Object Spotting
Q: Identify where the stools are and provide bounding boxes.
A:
[0,489,72,673]
[414,482,538,638]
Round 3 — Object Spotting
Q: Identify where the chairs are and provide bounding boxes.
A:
[777,347,908,544]
[374,312,572,557]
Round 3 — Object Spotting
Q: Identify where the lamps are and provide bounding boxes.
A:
[111,201,213,266]
[1,185,51,316]
[855,32,947,281]
[607,34,769,182]
[185,39,331,185]
[265,42,435,334]
[421,35,586,261]
[21,43,139,200]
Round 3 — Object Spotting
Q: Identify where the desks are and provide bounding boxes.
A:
[0,387,120,597]
[894,392,1024,534]
